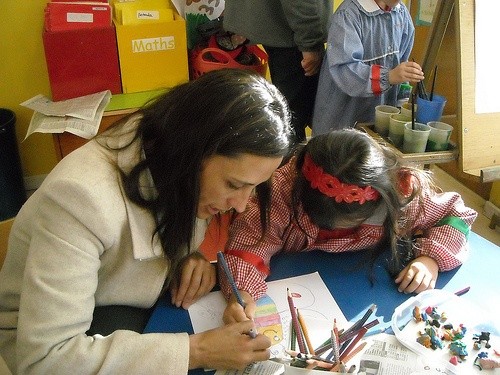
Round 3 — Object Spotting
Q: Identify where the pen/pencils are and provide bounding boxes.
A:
[455,287,470,296]
[249,328,256,338]
[217,250,246,309]
[286,287,381,374]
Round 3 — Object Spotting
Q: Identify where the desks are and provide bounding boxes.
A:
[51,87,173,160]
[143,230,499,375]
[357,122,461,172]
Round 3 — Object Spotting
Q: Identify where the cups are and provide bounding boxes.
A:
[416,94,448,124]
[400,102,417,117]
[403,122,432,154]
[387,113,412,152]
[374,104,400,139]
[426,121,454,152]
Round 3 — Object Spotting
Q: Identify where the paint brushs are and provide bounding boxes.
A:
[430,65,438,101]
[412,57,428,100]
[411,93,414,130]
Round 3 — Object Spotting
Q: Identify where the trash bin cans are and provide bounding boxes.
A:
[0,108,27,221]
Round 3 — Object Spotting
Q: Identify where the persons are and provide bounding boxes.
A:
[0,70,293,375]
[220,0,334,143]
[310,0,424,136]
[220,125,478,326]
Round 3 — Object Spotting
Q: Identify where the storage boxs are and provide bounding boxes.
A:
[42,0,190,100]
